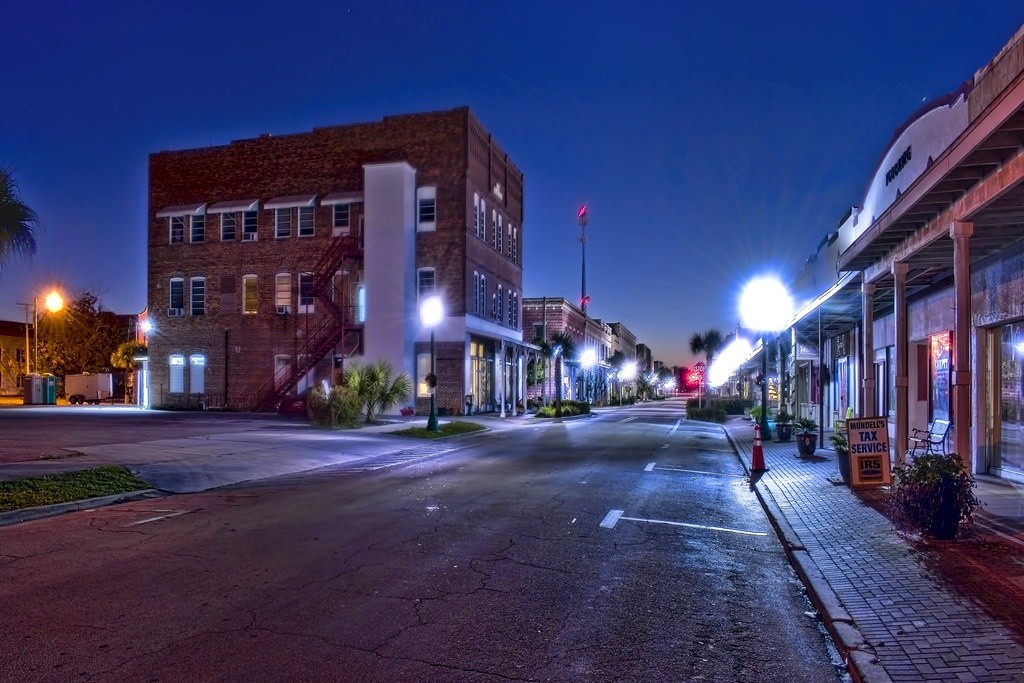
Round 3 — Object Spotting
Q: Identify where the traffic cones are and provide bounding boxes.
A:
[749,424,770,473]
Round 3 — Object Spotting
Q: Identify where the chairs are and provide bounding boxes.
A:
[833,407,852,432]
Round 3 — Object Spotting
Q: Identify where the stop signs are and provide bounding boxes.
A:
[693,362,707,376]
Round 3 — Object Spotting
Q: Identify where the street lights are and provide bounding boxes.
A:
[421,298,443,432]
[750,286,784,442]
[34,293,64,374]
[580,350,591,402]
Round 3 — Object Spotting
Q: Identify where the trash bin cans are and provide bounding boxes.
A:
[42,373,56,404]
[23,373,43,405]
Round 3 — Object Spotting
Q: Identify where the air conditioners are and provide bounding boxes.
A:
[277,306,286,314]
[168,308,183,316]
[243,233,258,241]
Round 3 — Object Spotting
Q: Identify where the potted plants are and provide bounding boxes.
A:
[774,411,795,441]
[886,455,986,542]
[830,433,849,481]
[794,416,818,456]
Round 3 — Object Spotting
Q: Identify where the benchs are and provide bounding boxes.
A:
[908,418,952,458]
